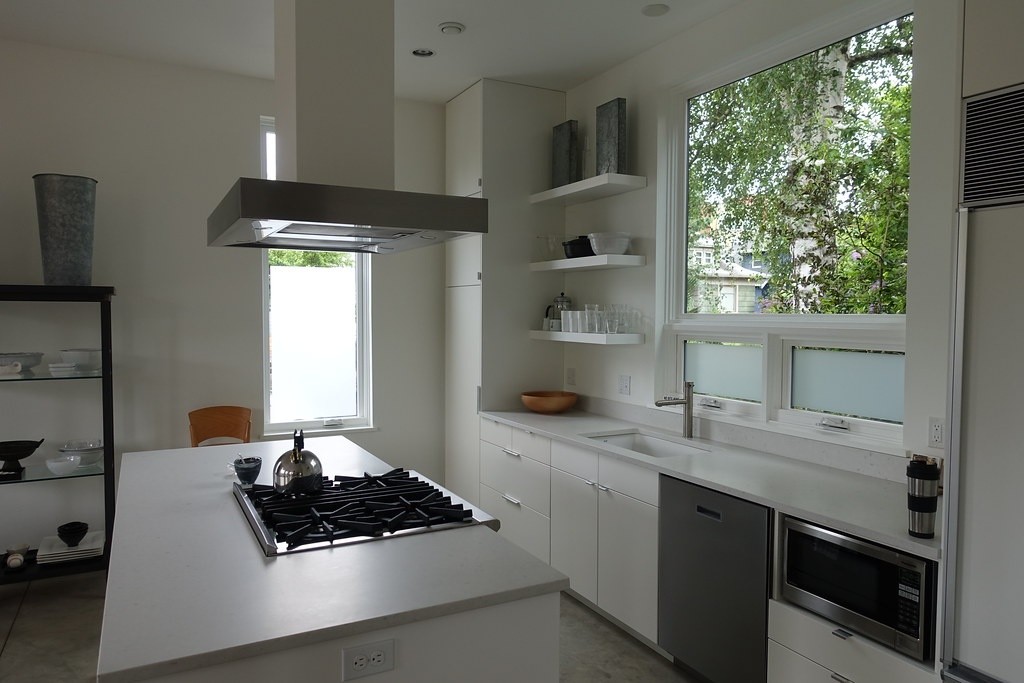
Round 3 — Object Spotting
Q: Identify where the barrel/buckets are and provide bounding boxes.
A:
[31,173,97,287]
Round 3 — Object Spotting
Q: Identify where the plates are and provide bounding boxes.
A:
[37,530,104,565]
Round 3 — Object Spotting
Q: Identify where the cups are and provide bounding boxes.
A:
[543,303,644,334]
[906,459,941,539]
[234,456,262,484]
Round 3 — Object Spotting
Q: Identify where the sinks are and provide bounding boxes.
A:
[576,427,712,458]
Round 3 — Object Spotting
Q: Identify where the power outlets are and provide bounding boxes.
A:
[341,636,394,681]
[928,416,944,448]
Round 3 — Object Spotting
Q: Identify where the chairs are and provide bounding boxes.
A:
[188,405,253,447]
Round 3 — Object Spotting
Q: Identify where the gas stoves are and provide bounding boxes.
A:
[233,467,478,557]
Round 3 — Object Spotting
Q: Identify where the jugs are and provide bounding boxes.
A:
[545,292,571,319]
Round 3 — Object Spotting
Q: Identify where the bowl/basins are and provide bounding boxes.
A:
[537,235,578,260]
[59,438,105,468]
[587,231,631,255]
[57,521,89,547]
[562,235,596,258]
[520,391,577,414]
[59,349,102,374]
[7,544,30,556]
[0,351,43,371]
[46,455,81,476]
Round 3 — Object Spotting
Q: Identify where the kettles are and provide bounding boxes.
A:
[273,429,324,497]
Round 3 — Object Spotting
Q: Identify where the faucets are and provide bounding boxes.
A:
[654,381,695,439]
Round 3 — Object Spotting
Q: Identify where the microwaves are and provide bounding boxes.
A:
[782,516,937,663]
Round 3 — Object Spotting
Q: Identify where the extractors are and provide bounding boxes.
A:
[204,0,492,257]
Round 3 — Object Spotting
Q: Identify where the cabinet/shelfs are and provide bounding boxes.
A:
[479,416,551,572]
[768,600,943,683]
[530,173,649,346]
[0,284,116,586]
[550,438,659,650]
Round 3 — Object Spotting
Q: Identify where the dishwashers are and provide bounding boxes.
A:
[654,474,773,683]
